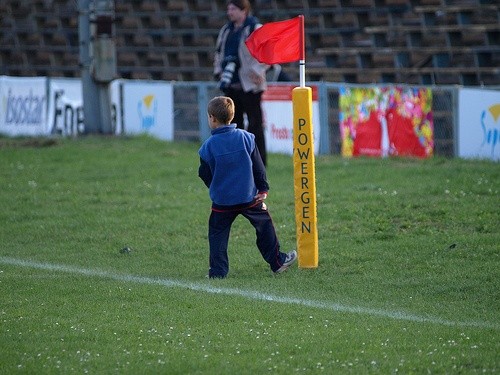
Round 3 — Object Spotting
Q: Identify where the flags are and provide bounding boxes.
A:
[244,15,305,66]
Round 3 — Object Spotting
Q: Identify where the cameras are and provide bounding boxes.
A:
[216,56,241,91]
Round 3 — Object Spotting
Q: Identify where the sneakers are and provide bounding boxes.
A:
[277,250,297,273]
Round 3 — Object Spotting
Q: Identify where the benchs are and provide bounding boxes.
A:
[0,1,498,158]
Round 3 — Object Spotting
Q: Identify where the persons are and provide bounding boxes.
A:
[198,96,298,280]
[212,0,272,167]
